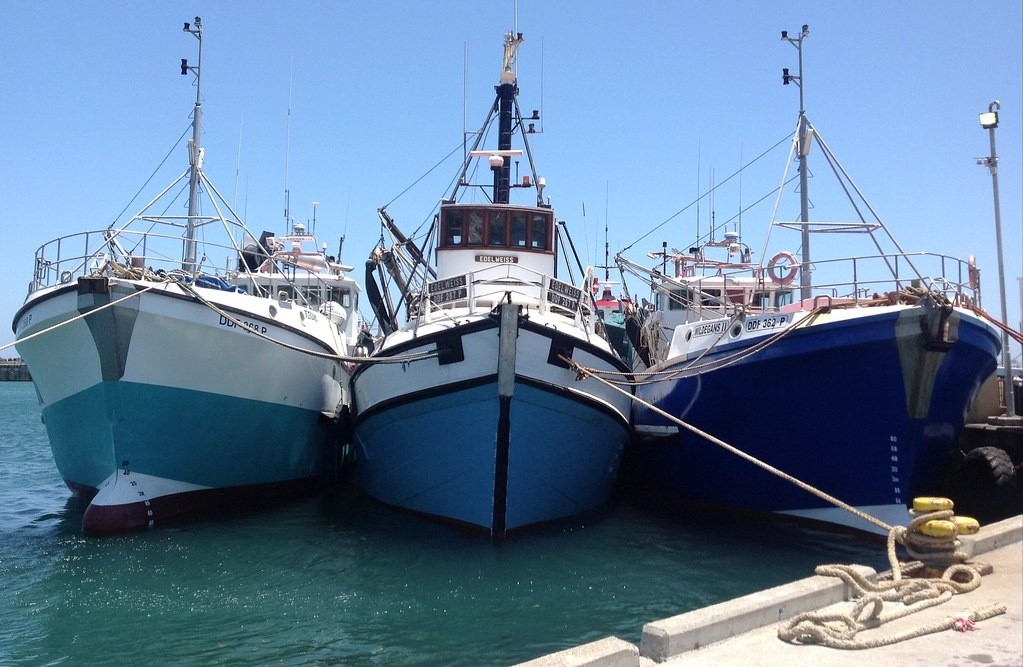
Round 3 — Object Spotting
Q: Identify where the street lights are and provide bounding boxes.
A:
[975,99,1023,426]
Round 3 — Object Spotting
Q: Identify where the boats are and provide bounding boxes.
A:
[10,14,374,541]
[344,157,798,380]
[596,17,1007,569]
[339,16,638,551]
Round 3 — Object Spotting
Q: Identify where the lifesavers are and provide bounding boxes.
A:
[961,445,1018,508]
[968,254,981,291]
[767,251,798,285]
